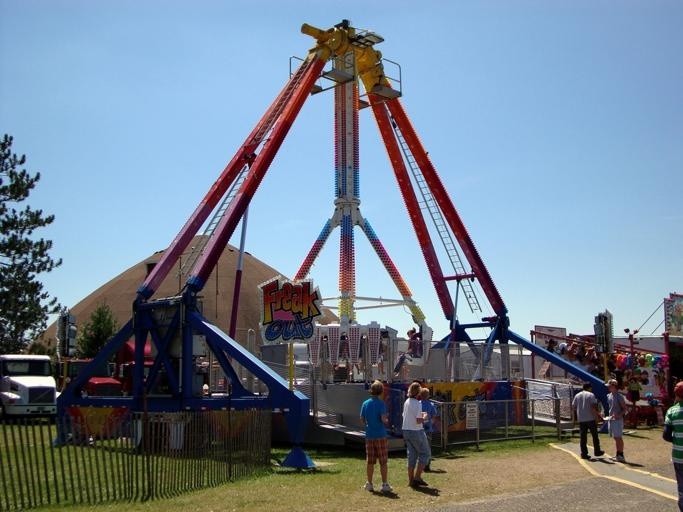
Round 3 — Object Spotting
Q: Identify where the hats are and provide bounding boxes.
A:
[673,381,683,397]
[605,379,618,387]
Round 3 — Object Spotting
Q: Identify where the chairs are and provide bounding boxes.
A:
[405,324,432,367]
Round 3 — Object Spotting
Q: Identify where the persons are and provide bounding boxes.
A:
[570,383,603,459]
[606,379,626,462]
[662,381,683,512]
[417,387,437,473]
[358,379,393,493]
[391,327,418,376]
[400,382,431,489]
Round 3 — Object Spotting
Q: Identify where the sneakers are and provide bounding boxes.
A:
[412,477,428,486]
[363,481,374,491]
[609,455,617,460]
[381,482,393,492]
[613,455,625,462]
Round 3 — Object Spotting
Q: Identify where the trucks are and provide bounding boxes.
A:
[0,353,64,421]
[110,337,156,395]
[56,359,122,400]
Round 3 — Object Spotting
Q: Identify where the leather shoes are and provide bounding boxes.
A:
[595,450,605,456]
[581,454,591,459]
